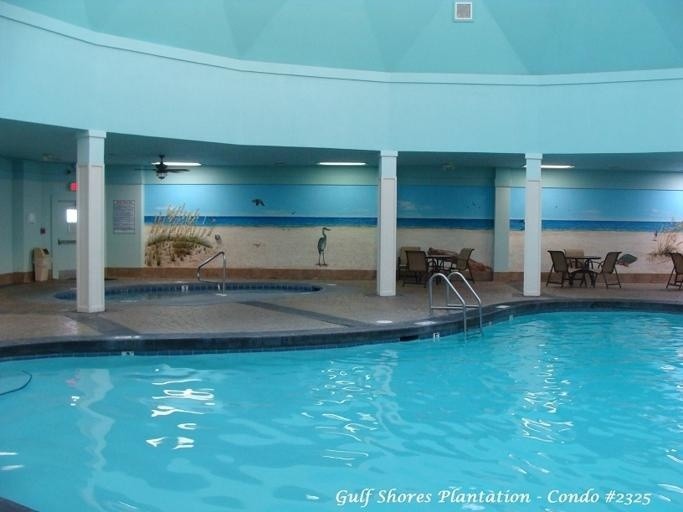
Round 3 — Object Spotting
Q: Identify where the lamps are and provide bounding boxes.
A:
[158,155,167,181]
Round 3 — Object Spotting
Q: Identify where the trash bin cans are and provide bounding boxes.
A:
[32,248,51,282]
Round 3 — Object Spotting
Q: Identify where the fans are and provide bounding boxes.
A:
[133,154,189,173]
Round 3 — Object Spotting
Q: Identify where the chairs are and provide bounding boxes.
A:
[546,248,637,289]
[399,246,475,289]
[665,252,683,290]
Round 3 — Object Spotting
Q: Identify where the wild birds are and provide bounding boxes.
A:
[252,198,266,207]
[317,227,330,265]
